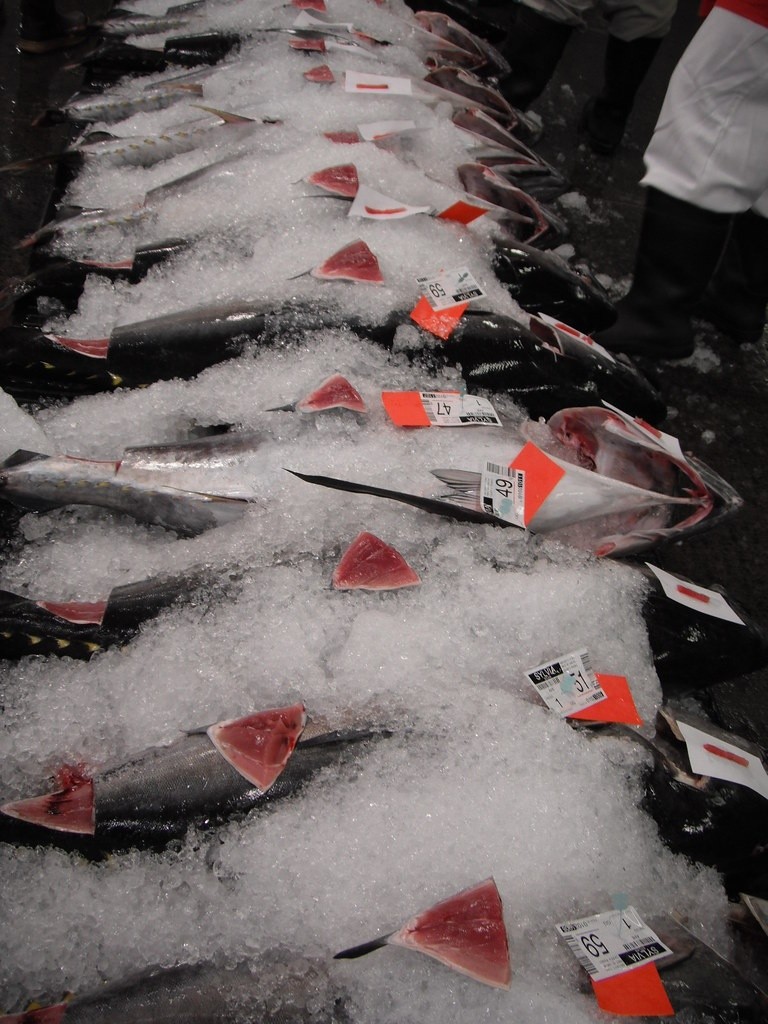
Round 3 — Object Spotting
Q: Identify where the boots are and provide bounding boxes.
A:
[491,2,577,114]
[691,208,768,343]
[579,33,664,154]
[584,186,737,361]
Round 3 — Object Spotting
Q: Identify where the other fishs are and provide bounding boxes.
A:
[0,0,768,1024]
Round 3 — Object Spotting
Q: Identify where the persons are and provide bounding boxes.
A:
[484,0,768,360]
